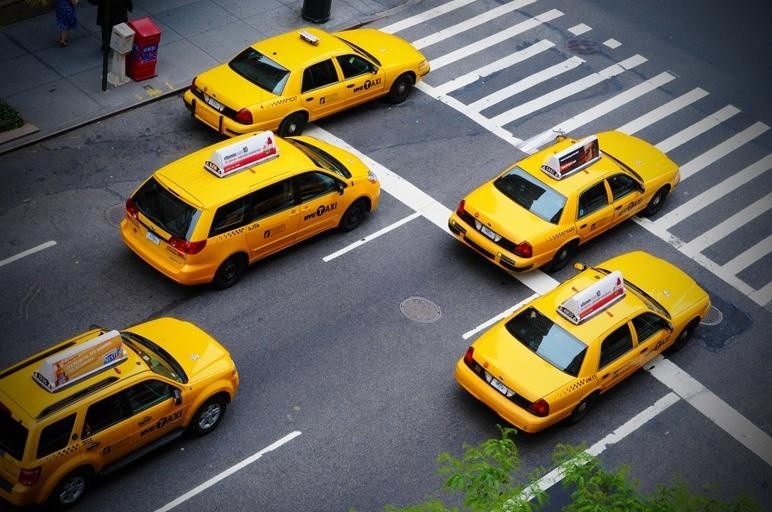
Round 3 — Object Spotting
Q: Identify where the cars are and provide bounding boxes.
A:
[180,24,434,144]
[1,308,245,512]
[119,128,385,294]
[451,248,713,437]
[444,128,685,280]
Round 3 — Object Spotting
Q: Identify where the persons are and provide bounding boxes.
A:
[579,140,598,162]
[88,0,134,54]
[55,1,80,48]
